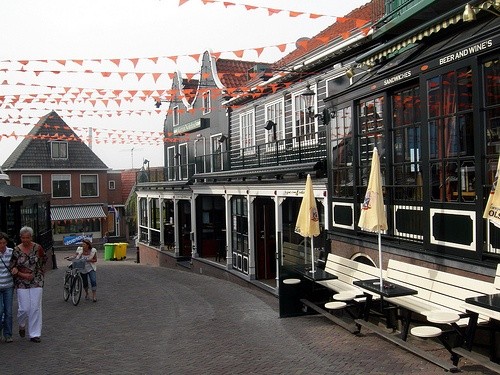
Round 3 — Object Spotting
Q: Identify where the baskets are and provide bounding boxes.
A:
[71,258,85,269]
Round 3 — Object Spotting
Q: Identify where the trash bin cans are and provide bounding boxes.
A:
[113,243,128,260]
[104,243,117,261]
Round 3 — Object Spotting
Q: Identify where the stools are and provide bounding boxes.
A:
[216,238,225,261]
[283,278,468,366]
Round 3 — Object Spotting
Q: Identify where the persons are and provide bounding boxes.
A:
[65,239,98,302]
[9,226,49,343]
[0,232,19,342]
[104,232,111,243]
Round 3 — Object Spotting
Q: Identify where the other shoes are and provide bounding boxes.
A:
[7,337,13,342]
[19,329,25,338]
[85,296,88,299]
[93,297,97,302]
[30,337,41,342]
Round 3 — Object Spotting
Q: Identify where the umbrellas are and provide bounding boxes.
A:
[294,174,321,271]
[358,146,388,286]
[481,157,500,229]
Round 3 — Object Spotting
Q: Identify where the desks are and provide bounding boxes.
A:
[465,293,500,313]
[296,266,338,298]
[352,279,419,316]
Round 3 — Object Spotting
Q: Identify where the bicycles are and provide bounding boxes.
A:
[63,257,87,306]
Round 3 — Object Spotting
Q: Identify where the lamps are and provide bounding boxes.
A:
[174,152,181,164]
[155,99,177,108]
[462,2,500,21]
[345,65,373,78]
[222,90,253,101]
[264,120,277,139]
[301,82,330,126]
[218,135,227,151]
[144,159,150,170]
[260,67,299,81]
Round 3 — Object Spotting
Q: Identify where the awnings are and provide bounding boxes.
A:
[50,205,107,225]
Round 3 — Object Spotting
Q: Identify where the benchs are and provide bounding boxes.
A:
[316,253,500,359]
